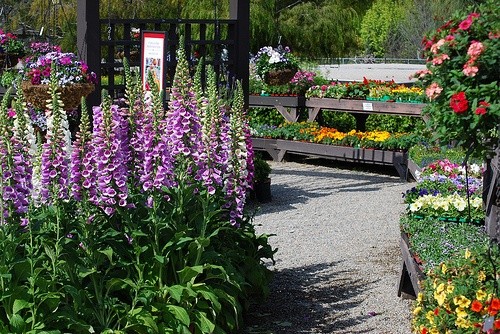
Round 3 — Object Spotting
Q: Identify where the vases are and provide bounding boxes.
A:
[21,83,96,114]
[258,68,298,86]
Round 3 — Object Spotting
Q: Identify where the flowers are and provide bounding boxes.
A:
[248,45,301,83]
[12,51,98,85]
